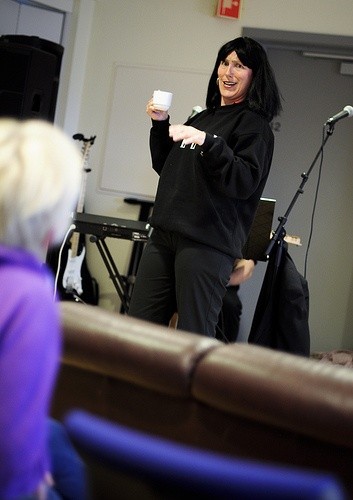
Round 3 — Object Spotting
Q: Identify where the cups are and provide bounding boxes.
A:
[150,90,172,111]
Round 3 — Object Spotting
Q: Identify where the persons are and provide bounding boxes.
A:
[216,248,310,356]
[0,118,84,500]
[129,36,288,339]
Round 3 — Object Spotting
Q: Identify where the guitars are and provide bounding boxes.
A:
[55,133,99,305]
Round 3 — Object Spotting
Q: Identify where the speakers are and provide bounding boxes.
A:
[0,35,64,125]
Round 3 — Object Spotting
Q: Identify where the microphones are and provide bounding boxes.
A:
[325,105,353,126]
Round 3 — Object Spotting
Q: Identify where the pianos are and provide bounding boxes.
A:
[72,212,155,319]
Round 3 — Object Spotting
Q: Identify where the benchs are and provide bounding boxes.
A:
[49,300,353,500]
